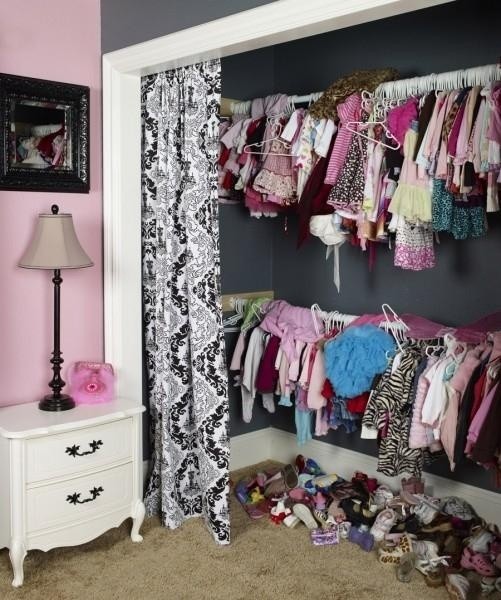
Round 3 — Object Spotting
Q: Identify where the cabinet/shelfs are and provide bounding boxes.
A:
[0,393,147,588]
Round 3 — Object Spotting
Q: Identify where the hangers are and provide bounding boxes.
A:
[232,91,323,126]
[244,123,292,157]
[380,302,468,362]
[360,65,501,117]
[310,302,358,335]
[222,298,266,333]
[345,109,400,152]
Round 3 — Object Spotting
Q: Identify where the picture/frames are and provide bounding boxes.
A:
[1,71,92,195]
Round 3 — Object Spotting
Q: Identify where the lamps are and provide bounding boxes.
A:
[16,204,95,410]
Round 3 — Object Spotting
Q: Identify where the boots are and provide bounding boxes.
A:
[349,527,374,552]
[378,531,413,567]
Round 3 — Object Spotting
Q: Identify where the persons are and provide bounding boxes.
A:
[28,126,65,164]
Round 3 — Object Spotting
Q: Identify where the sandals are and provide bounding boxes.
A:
[313,508,337,530]
[461,548,495,577]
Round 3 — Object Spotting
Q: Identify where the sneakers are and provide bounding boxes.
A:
[397,552,418,583]
[293,503,318,529]
[478,577,495,597]
[370,508,395,544]
[445,499,471,521]
[413,540,438,559]
[445,573,470,600]
[411,500,438,524]
[426,563,463,587]
[283,462,299,489]
[283,514,300,530]
[416,555,438,577]
